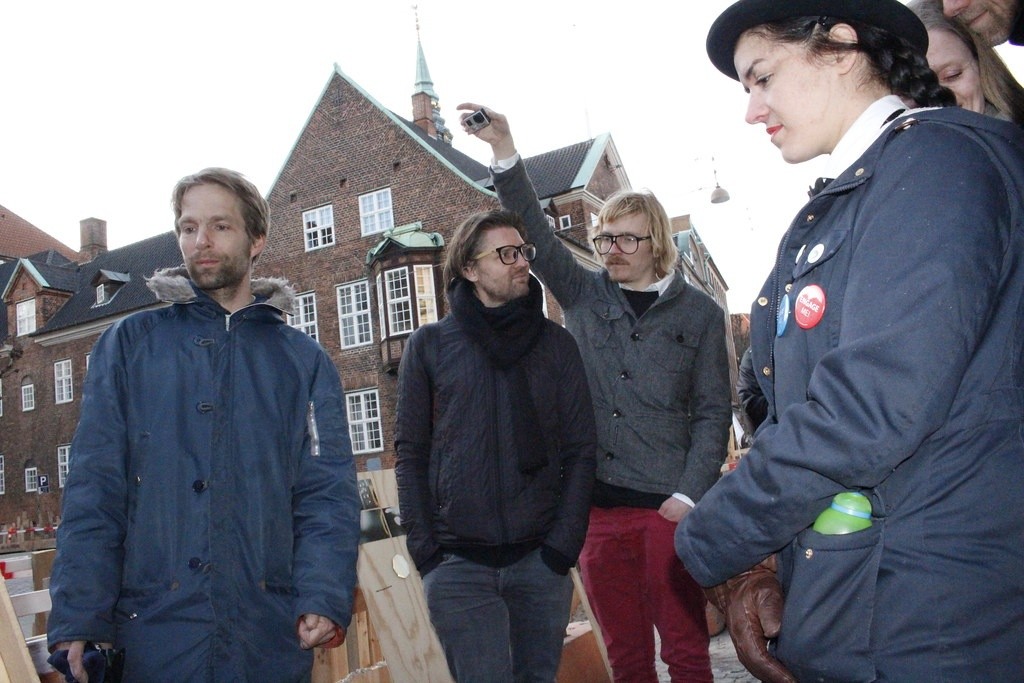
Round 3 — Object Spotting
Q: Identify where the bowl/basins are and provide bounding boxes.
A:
[358,507,387,542]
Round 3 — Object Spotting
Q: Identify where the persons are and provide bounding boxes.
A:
[672,0,1024,683]
[45,166,362,683]
[395,103,734,683]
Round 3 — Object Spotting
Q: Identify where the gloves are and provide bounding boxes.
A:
[701,553,799,683]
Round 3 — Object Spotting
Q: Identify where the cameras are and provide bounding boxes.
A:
[464,108,491,132]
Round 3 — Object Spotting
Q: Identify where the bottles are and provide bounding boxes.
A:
[812,491,871,534]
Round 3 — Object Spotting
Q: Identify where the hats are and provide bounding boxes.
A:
[706,0,929,82]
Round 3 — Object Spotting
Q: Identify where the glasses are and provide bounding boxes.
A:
[472,242,537,265]
[593,234,651,255]
